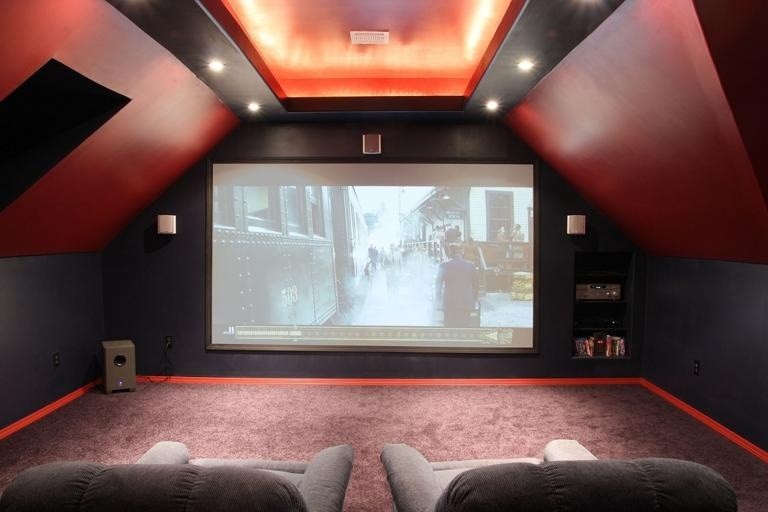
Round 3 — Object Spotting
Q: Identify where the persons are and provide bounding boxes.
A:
[432,241,481,329]
[359,222,526,293]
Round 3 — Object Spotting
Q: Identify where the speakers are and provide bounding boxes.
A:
[362,134,382,154]
[100,339,136,393]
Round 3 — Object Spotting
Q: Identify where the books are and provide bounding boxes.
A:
[571,333,628,358]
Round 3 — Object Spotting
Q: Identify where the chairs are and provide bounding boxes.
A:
[378,437,737,509]
[1,441,354,510]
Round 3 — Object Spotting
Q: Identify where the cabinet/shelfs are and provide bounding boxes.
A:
[569,239,639,363]
